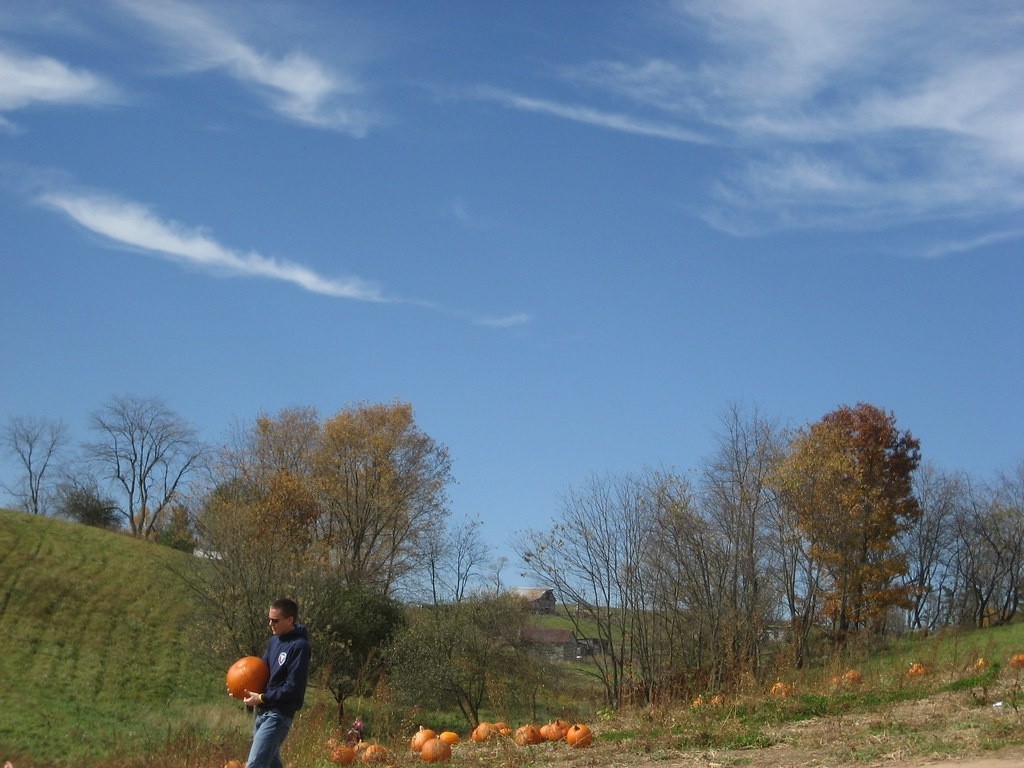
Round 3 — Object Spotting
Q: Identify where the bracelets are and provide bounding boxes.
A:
[259,694,264,703]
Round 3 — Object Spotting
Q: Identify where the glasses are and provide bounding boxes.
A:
[268,615,293,624]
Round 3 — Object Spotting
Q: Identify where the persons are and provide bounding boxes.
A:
[225,598,312,768]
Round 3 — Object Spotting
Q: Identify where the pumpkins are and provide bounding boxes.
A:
[227,656,269,699]
[325,737,388,764]
[691,652,1024,707]
[221,760,243,768]
[410,719,592,762]
